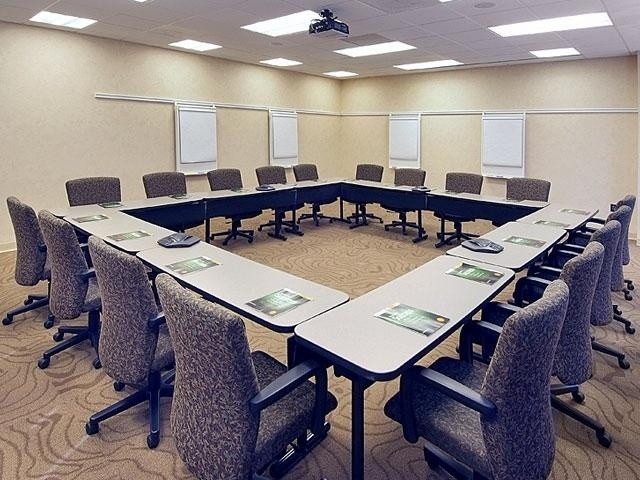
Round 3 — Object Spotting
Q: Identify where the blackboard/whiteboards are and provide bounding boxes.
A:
[481,112,526,180]
[268,107,300,169]
[173,101,218,176]
[388,113,421,170]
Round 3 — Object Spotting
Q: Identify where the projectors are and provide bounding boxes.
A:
[306,19,350,40]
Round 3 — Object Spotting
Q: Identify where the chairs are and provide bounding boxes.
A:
[0,196,84,330]
[153,271,339,479]
[142,171,187,199]
[380,168,427,237]
[292,163,333,227]
[432,171,484,248]
[526,220,631,372]
[554,205,636,335]
[579,194,637,301]
[492,177,551,228]
[346,164,384,224]
[206,167,255,246]
[84,235,176,451]
[384,280,571,479]
[255,165,300,232]
[65,176,122,207]
[36,210,101,370]
[481,241,613,450]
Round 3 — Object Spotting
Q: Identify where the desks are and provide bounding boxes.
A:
[286,177,352,237]
[63,208,177,254]
[293,254,517,480]
[188,183,294,241]
[515,202,600,231]
[445,221,566,274]
[48,195,205,232]
[340,179,438,244]
[135,239,352,417]
[425,189,550,248]
